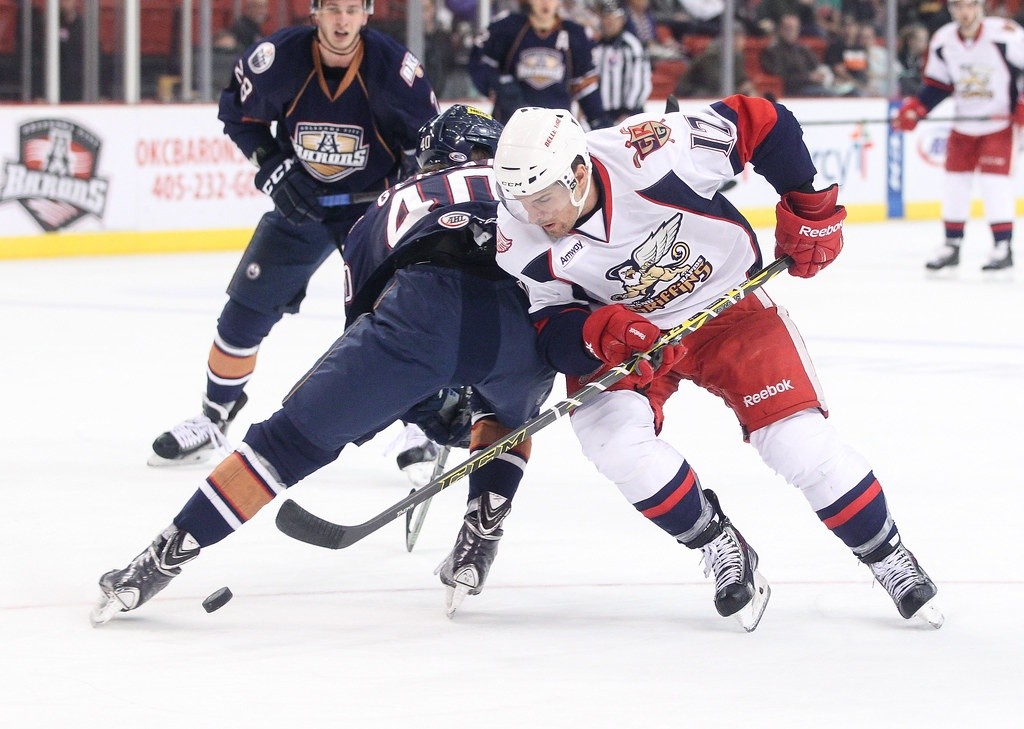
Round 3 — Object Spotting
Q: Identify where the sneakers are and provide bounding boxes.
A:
[90,522,199,627]
[925,239,1015,277]
[147,390,248,470]
[395,418,446,486]
[433,490,512,620]
[677,489,771,632]
[852,520,945,630]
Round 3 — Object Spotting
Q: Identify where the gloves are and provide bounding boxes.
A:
[583,304,691,388]
[255,153,328,229]
[890,97,927,133]
[1012,94,1024,127]
[490,69,524,109]
[420,385,475,449]
[775,182,847,279]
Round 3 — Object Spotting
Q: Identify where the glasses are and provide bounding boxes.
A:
[468,145,494,167]
[310,1,375,16]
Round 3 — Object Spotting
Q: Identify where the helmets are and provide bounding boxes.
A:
[947,0,984,35]
[415,103,504,169]
[494,105,593,207]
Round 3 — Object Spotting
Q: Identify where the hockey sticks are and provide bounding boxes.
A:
[401,444,453,553]
[276,253,795,550]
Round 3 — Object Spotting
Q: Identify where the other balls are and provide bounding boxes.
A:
[202,587,233,614]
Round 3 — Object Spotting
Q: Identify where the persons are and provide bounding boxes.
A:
[90,104,554,626]
[16,0,1024,466]
[494,93,945,627]
